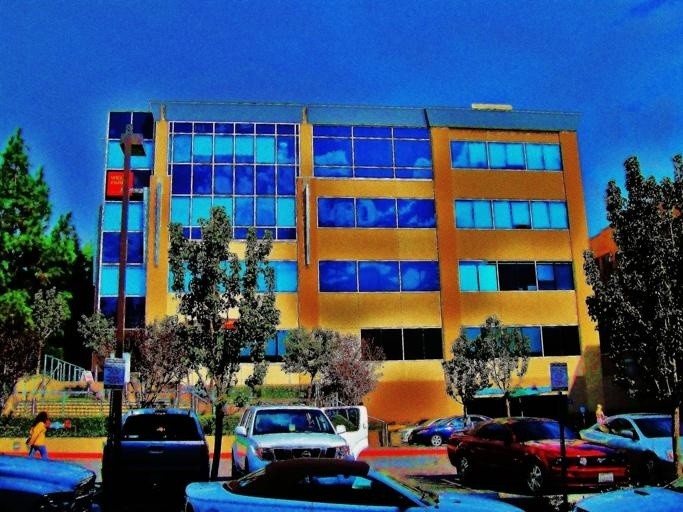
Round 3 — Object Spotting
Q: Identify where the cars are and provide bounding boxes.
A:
[96,407,214,500]
[177,456,528,512]
[0,456,100,512]
[398,406,681,512]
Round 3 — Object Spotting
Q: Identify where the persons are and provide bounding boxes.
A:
[25,411,48,460]
[594,402,607,425]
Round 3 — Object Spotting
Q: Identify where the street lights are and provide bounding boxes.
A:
[106,126,149,447]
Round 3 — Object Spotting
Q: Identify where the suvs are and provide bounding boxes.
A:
[225,401,371,483]
